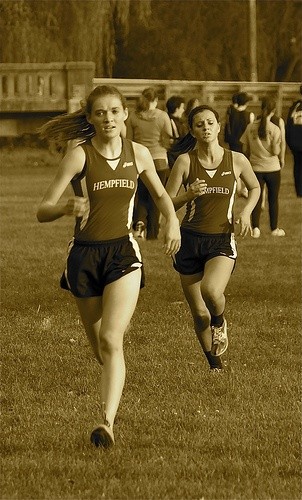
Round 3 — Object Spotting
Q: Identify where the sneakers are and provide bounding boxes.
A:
[210,318,228,356]
[207,367,224,375]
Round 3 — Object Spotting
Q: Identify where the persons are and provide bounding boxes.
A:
[35,85,182,448]
[164,104,261,372]
[123,82,302,242]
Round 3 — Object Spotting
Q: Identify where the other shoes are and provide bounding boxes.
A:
[89,424,114,452]
[240,190,248,198]
[135,221,146,240]
[272,228,285,236]
[252,227,260,238]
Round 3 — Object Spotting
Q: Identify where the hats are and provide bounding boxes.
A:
[237,93,252,104]
